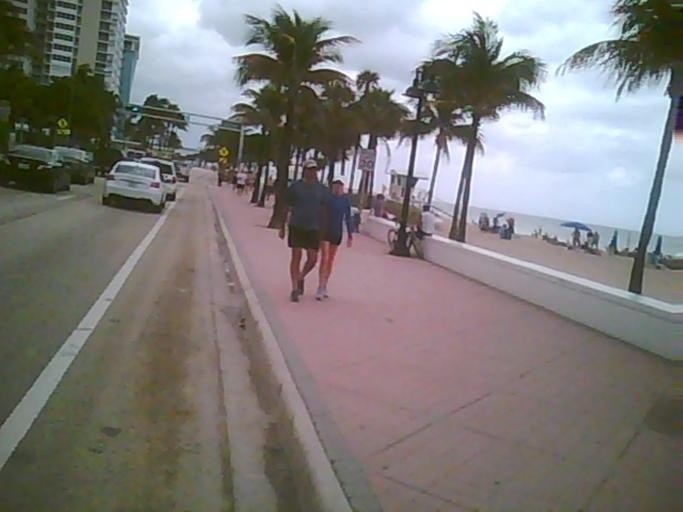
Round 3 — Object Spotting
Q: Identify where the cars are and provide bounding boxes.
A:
[140,156,178,201]
[103,160,166,210]
[0,145,71,193]
[52,141,191,183]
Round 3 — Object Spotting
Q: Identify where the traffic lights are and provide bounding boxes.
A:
[124,106,143,113]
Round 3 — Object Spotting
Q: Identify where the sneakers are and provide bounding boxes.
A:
[290,280,329,301]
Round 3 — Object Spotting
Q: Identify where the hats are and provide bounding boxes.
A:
[302,159,318,169]
[332,177,344,185]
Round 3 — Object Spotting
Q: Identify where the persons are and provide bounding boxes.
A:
[474,212,663,260]
[373,193,384,218]
[314,177,352,301]
[218,165,265,200]
[407,203,435,260]
[276,159,328,302]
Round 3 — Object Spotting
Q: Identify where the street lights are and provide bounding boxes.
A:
[14,116,31,144]
[389,68,445,259]
